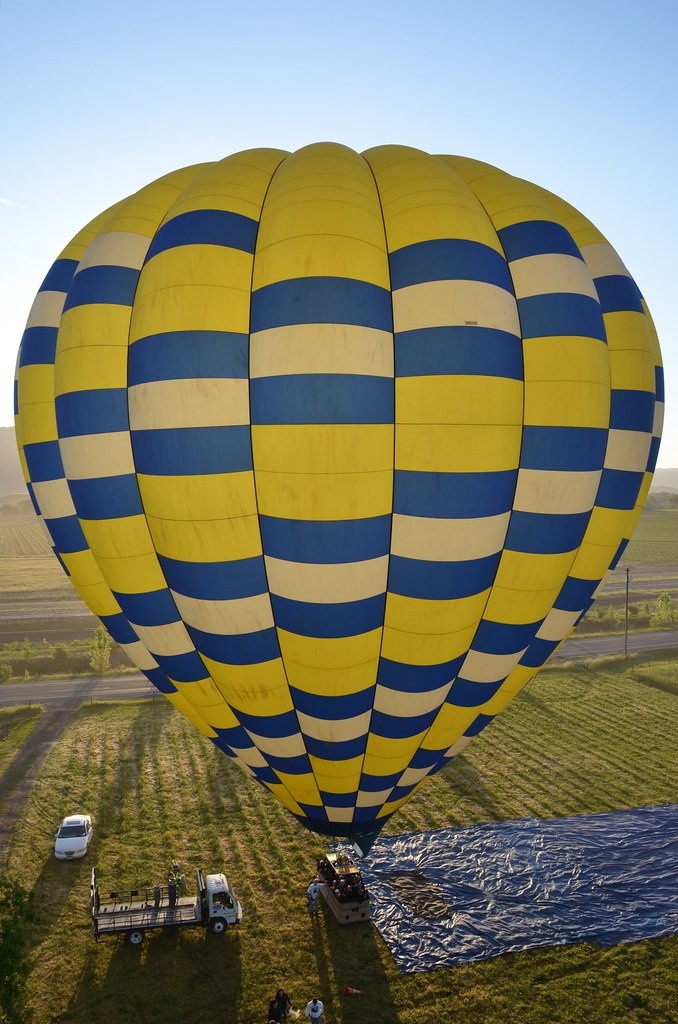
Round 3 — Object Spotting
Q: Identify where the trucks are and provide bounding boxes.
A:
[91,870,242,946]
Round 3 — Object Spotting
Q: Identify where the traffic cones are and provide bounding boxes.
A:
[343,985,364,996]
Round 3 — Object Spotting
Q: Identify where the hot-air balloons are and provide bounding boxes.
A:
[9,141,668,923]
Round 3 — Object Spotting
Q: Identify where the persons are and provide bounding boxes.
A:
[307,875,327,908]
[275,988,292,1020]
[307,880,328,917]
[268,1000,282,1024]
[318,860,369,904]
[306,999,324,1024]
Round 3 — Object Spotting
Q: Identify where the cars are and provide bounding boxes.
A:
[53,812,92,861]
